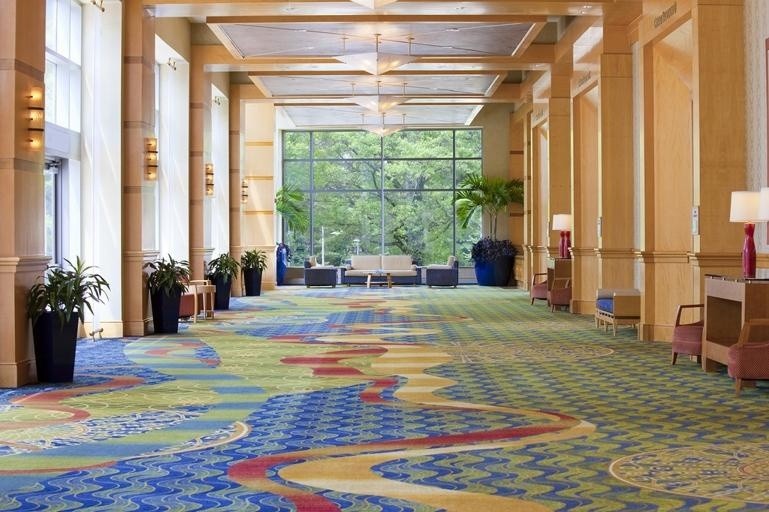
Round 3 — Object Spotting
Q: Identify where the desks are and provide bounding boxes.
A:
[187,285,216,320]
[546,258,571,311]
[701,276,769,373]
[363,272,394,288]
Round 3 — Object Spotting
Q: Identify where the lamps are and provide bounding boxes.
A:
[551,214,572,258]
[206,163,215,198]
[333,0,421,137]
[729,187,769,279]
[26,86,45,152]
[144,138,158,181]
[242,180,249,203]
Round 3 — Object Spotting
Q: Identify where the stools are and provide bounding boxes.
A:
[595,288,640,337]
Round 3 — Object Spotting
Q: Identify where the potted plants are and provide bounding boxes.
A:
[276,184,307,286]
[28,256,111,385]
[241,248,267,297]
[208,253,241,310]
[146,253,193,334]
[450,172,524,286]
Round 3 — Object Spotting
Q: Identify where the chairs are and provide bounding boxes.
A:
[304,256,337,288]
[671,304,769,397]
[340,256,421,287]
[425,256,459,288]
[178,279,211,323]
[531,273,572,312]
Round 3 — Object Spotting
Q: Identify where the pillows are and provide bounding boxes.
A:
[309,256,317,268]
[351,256,412,271]
[447,256,455,266]
